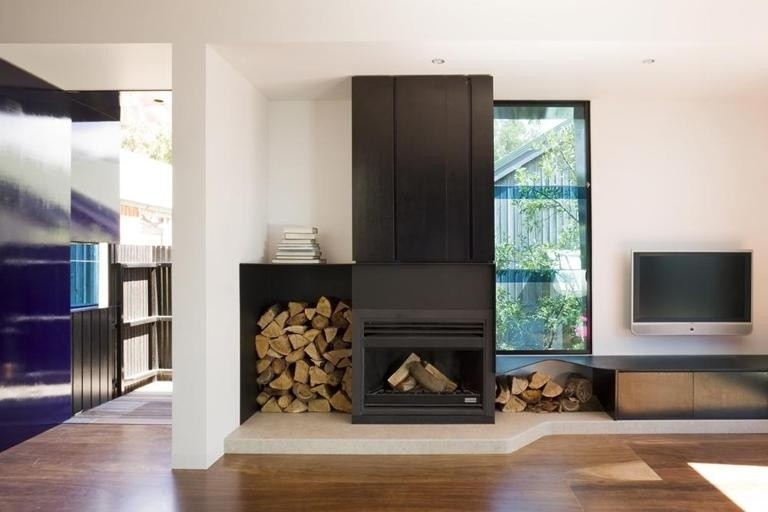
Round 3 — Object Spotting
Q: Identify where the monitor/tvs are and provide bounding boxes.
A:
[631,250,753,335]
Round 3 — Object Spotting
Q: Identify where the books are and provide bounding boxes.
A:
[271,228,326,264]
